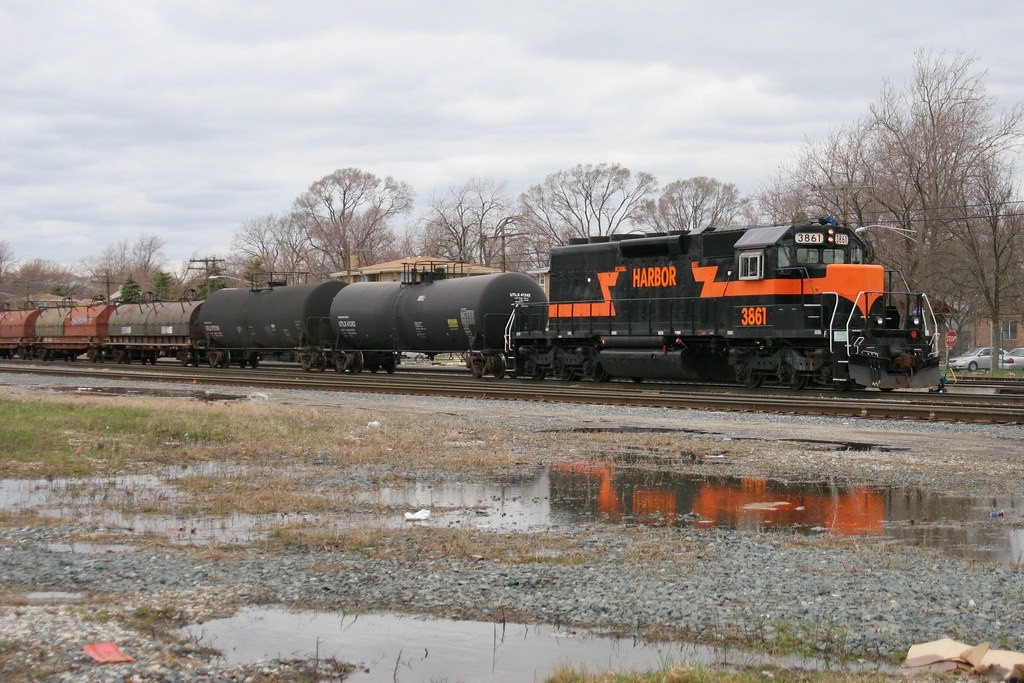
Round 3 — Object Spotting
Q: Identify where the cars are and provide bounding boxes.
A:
[998,348,1024,373]
[945,347,1009,372]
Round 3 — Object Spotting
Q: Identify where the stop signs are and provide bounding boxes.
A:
[945,331,958,350]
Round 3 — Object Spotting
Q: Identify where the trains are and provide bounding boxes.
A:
[1,216,947,395]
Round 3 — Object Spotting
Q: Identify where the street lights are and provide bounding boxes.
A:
[852,224,932,332]
